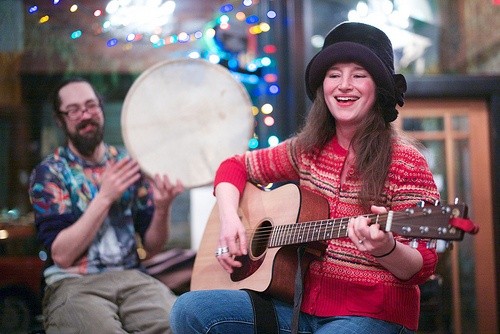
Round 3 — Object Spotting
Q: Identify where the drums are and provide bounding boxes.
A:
[119,57,256,190]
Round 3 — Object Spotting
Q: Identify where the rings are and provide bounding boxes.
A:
[216,248,221,257]
[221,247,228,254]
[371,239,396,258]
[359,236,366,244]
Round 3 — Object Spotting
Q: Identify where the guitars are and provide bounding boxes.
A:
[189,178,479,301]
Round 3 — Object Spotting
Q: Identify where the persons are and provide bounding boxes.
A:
[30,78,182,334]
[167,22,453,334]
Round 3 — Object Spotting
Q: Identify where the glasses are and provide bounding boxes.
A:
[59,101,103,121]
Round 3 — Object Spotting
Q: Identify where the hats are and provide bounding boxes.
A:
[305,21,407,122]
